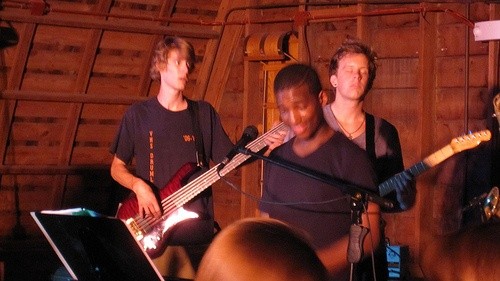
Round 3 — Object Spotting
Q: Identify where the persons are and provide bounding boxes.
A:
[255,62,381,281]
[107,34,288,276]
[318,39,417,281]
[193,216,331,281]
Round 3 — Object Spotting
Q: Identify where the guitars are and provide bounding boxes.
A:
[375,127,494,198]
[115,121,290,260]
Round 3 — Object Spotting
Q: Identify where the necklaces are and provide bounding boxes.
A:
[333,109,367,141]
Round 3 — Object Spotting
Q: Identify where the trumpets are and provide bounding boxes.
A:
[480,92,500,224]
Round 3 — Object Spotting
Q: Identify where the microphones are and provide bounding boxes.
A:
[217,125,258,171]
